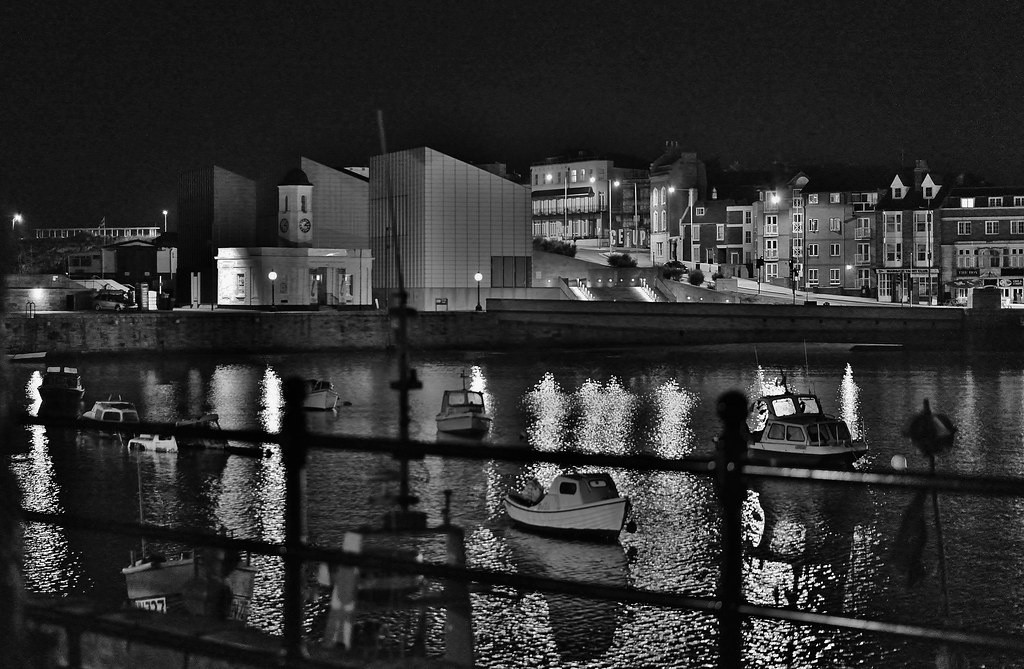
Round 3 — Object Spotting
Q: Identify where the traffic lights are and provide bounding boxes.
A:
[789,268,794,282]
[757,259,766,270]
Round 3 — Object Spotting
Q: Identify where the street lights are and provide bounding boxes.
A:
[668,187,695,270]
[613,181,639,252]
[772,195,807,292]
[546,172,567,240]
[268,268,277,313]
[474,272,484,311]
[588,177,613,254]
[163,209,168,232]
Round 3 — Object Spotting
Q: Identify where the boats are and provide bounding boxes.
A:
[502,470,639,541]
[435,368,492,439]
[38,366,89,404]
[4,336,49,359]
[711,341,873,481]
[302,381,341,412]
[74,392,233,455]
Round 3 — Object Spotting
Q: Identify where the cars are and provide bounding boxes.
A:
[88,294,139,312]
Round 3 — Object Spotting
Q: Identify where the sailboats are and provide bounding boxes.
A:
[123,441,200,603]
